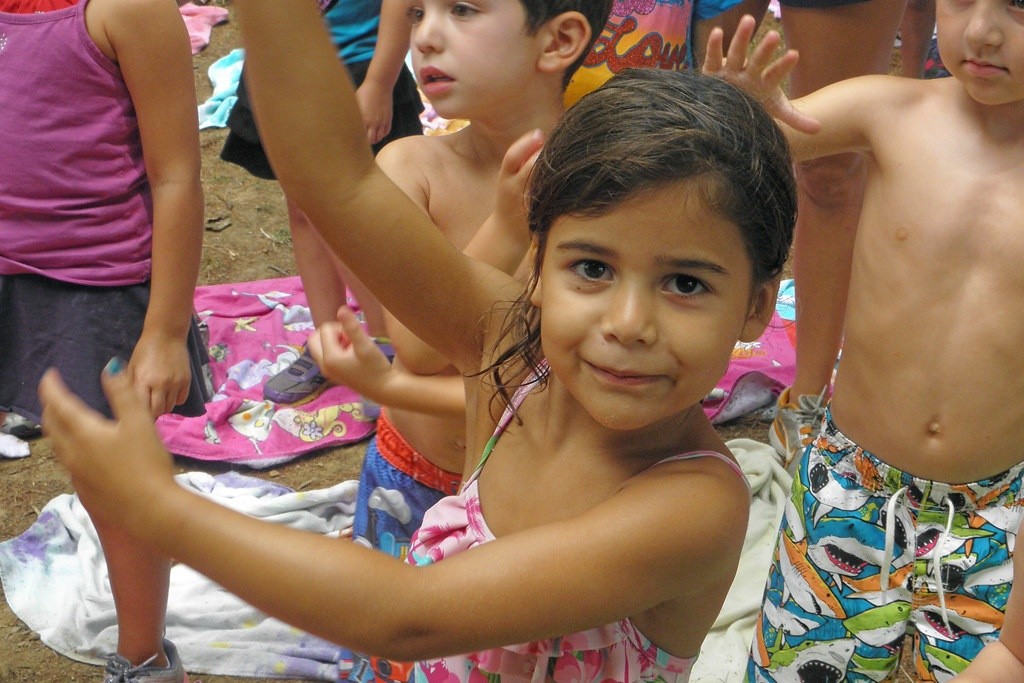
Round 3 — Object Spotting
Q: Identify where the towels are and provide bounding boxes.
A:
[685,434,797,682]
[143,276,821,473]
[197,43,247,129]
[1,471,361,677]
[173,3,230,57]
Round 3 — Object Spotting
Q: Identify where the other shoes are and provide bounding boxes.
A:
[0,411,52,460]
[923,37,953,80]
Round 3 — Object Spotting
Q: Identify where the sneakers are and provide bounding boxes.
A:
[103,639,184,682]
[373,337,398,365]
[770,385,831,477]
[263,346,331,407]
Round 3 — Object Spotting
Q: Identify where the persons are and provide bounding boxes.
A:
[42,0,797,682]
[0,0,1023,683]
